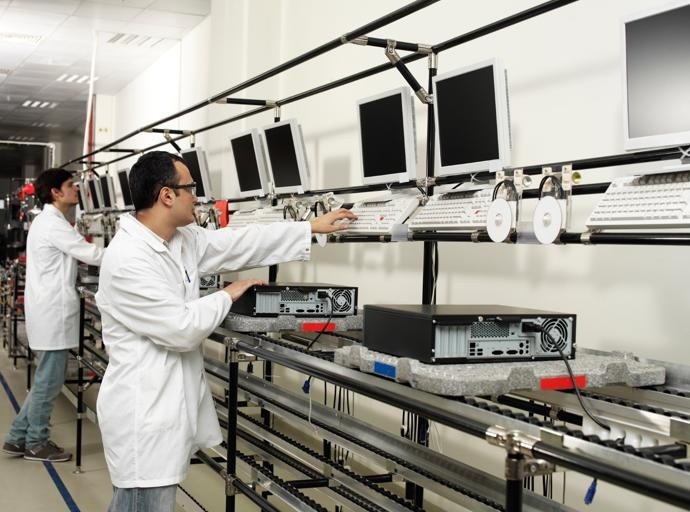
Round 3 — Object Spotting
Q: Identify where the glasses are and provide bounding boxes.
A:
[159,179,197,196]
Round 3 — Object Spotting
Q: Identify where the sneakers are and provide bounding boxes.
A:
[3,437,72,463]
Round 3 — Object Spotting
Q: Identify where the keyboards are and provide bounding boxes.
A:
[222,211,290,231]
[586,172,690,235]
[412,198,493,228]
[336,206,406,233]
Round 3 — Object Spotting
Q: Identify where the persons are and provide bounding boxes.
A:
[93,149,359,512]
[1,166,107,463]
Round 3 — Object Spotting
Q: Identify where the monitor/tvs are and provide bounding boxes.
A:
[260,118,307,194]
[356,85,413,184]
[621,3,690,152]
[432,60,511,176]
[76,149,211,206]
[229,128,266,197]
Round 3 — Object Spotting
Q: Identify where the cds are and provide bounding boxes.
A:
[533,195,563,245]
[317,232,330,248]
[487,198,512,242]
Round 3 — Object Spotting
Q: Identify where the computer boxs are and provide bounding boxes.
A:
[362,302,578,366]
[224,278,359,318]
